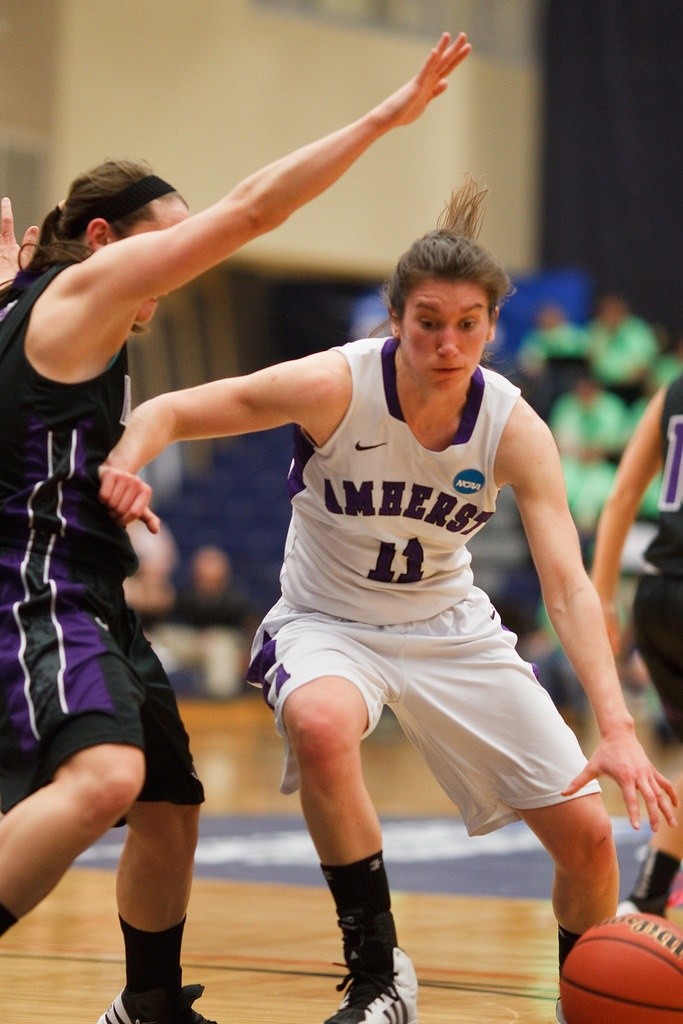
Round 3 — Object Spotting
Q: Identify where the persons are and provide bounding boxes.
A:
[127,269,683,712]
[592,369,683,919]
[98,174,679,1024]
[0,31,472,1024]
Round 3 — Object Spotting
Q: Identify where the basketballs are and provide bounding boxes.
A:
[556,911,683,1024]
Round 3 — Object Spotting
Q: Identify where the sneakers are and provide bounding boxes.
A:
[322,942,418,1024]
[97,978,216,1024]
